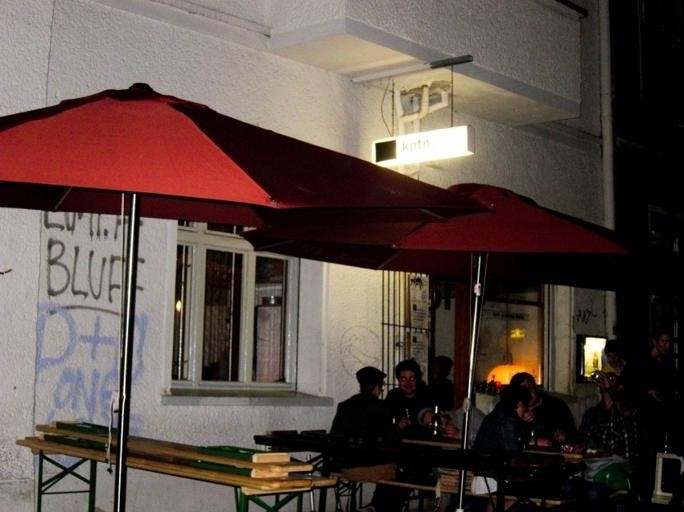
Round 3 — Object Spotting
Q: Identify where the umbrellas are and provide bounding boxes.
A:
[0,81,501,510]
[238,179,659,510]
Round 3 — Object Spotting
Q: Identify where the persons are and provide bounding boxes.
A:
[326,366,418,511]
[598,339,626,377]
[415,391,484,511]
[509,371,576,451]
[385,357,441,434]
[619,329,682,418]
[560,370,655,511]
[430,354,455,411]
[472,382,538,511]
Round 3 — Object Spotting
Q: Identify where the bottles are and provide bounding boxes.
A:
[430,403,442,442]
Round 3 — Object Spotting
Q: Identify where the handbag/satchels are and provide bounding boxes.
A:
[582,456,631,490]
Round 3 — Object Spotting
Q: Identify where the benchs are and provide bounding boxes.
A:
[339,478,564,512]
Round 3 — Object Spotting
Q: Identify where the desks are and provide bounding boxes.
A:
[402,438,596,512]
[16,423,338,512]
